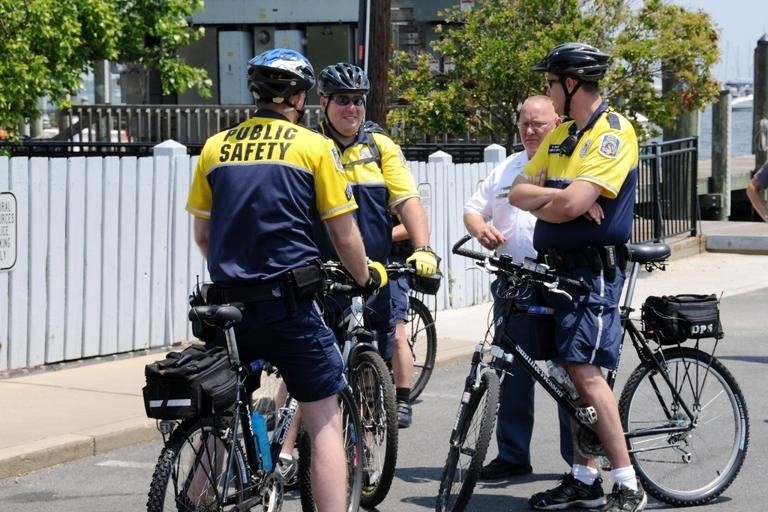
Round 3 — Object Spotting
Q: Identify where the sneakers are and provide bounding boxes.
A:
[600,476,648,512]
[476,455,533,486]
[528,469,608,511]
[397,401,413,429]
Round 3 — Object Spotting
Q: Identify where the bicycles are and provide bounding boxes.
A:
[434,232,751,511]
[314,249,434,512]
[146,278,381,512]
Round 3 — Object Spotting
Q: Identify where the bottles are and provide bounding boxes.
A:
[544,358,581,402]
[250,408,274,474]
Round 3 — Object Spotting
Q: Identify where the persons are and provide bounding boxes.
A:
[508,42,650,512]
[183,48,388,512]
[463,96,574,481]
[389,209,413,428]
[746,160,768,224]
[270,62,439,492]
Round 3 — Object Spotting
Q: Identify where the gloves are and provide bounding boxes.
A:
[405,246,438,278]
[365,261,389,294]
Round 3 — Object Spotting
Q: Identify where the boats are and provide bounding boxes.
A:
[732,94,753,112]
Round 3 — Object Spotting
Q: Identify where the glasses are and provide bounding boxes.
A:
[331,95,364,106]
[517,118,558,129]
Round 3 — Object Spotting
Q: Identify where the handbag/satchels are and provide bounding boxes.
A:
[640,291,725,345]
[142,343,238,420]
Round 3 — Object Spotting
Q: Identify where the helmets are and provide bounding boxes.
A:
[246,48,317,104]
[530,42,611,82]
[316,63,371,97]
[363,121,384,135]
